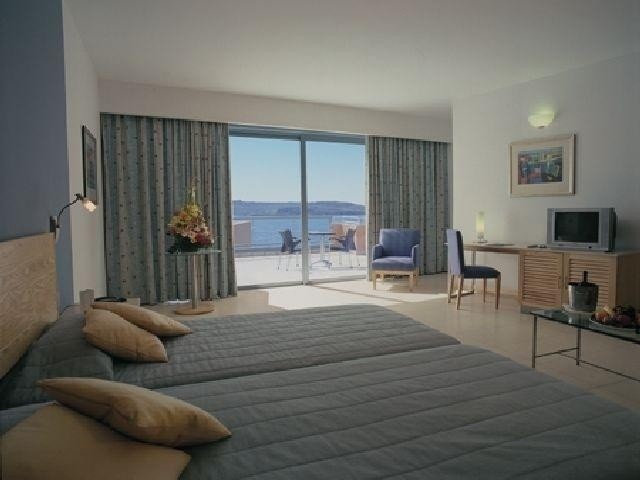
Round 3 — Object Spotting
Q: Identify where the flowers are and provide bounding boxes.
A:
[165,200,216,253]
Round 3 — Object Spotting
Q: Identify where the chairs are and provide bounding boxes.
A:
[331,227,361,270]
[276,230,301,271]
[369,227,422,293]
[446,228,502,311]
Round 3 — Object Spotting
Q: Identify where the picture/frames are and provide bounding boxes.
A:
[507,133,575,198]
[81,124,99,207]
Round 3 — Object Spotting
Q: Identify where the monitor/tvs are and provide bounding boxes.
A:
[546,207,616,253]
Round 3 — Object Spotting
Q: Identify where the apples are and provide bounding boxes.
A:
[594,303,640,328]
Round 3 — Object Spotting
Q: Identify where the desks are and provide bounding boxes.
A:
[443,242,640,317]
[309,231,337,267]
[169,249,222,317]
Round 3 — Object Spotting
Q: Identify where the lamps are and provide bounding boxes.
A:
[473,211,488,244]
[49,194,97,242]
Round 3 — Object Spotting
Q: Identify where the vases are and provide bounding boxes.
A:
[179,238,199,253]
